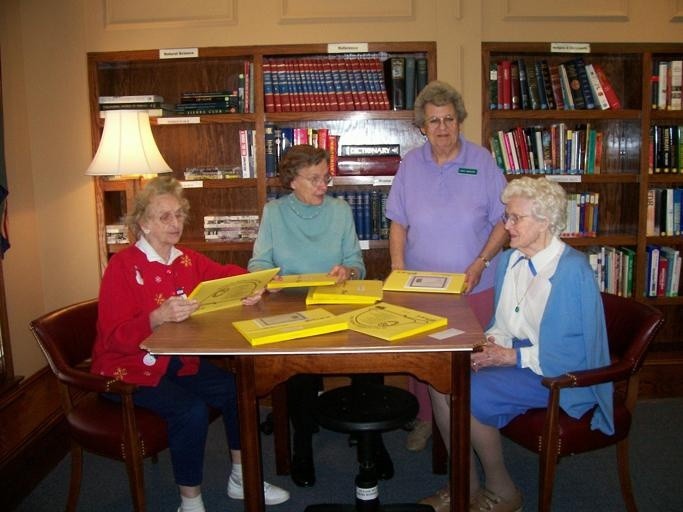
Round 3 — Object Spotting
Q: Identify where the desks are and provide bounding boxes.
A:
[139,287,487,512]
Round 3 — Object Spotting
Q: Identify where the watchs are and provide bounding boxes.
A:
[475,254,490,269]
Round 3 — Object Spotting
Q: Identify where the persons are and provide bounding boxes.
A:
[243,141,397,490]
[378,79,511,456]
[414,172,620,512]
[82,173,294,512]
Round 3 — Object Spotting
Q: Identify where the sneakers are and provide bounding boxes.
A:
[227,475,290,505]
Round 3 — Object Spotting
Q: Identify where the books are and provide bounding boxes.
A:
[649,124,681,174]
[238,61,254,114]
[647,188,681,236]
[581,245,635,298]
[204,215,259,242]
[268,192,391,240]
[652,60,682,111]
[106,225,130,244]
[560,192,599,239]
[184,130,257,181]
[489,122,602,175]
[488,59,622,110]
[263,52,426,113]
[646,245,682,296]
[99,90,238,118]
[265,122,401,177]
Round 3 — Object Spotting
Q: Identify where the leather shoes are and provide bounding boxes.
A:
[291,431,315,488]
[417,481,526,512]
[406,421,432,452]
[358,432,394,480]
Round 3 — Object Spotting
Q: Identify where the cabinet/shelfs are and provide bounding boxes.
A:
[87,42,441,402]
[477,43,682,402]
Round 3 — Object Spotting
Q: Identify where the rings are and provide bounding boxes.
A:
[472,360,476,367]
[180,292,189,300]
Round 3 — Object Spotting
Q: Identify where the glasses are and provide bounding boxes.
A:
[309,173,332,185]
[501,213,534,224]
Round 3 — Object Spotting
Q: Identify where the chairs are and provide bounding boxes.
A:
[28,298,171,511]
[498,291,666,512]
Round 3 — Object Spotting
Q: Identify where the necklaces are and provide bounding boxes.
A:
[510,262,536,313]
